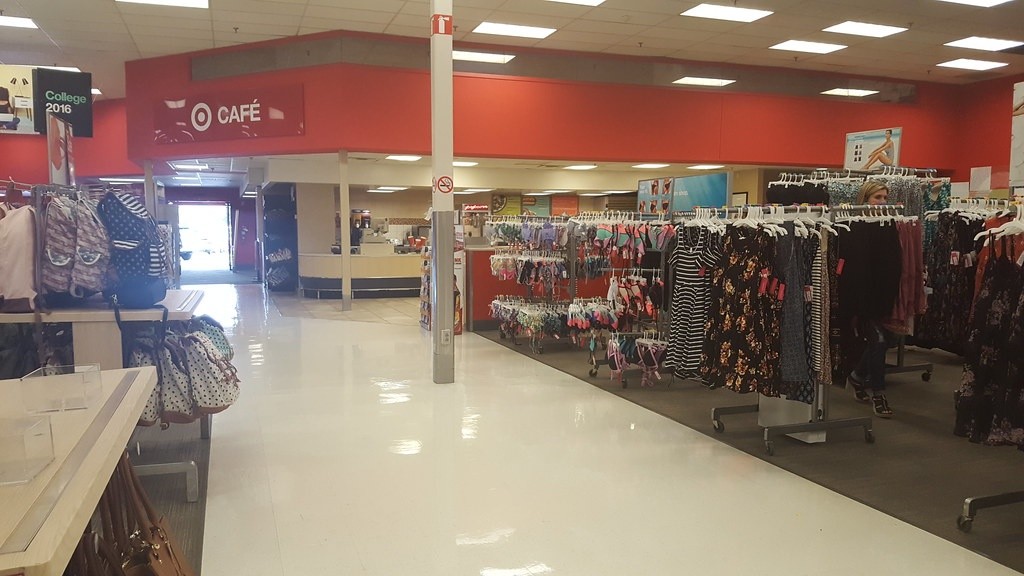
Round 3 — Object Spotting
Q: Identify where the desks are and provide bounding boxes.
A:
[0,366,159,576]
[0,290,211,503]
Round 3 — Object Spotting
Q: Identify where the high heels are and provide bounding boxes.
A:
[845,377,894,419]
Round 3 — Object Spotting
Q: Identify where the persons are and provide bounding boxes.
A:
[845,181,896,418]
[861,130,894,170]
[639,178,670,214]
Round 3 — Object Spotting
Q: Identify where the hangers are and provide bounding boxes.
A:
[490,247,566,263]
[609,267,661,283]
[768,166,951,188]
[492,294,613,322]
[924,198,1024,246]
[484,204,918,239]
[608,331,669,350]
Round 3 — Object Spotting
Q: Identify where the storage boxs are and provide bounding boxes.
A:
[20,363,102,415]
[0,416,56,486]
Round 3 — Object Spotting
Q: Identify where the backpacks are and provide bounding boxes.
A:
[0,189,245,430]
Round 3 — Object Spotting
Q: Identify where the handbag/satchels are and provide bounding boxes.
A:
[67,506,196,576]
